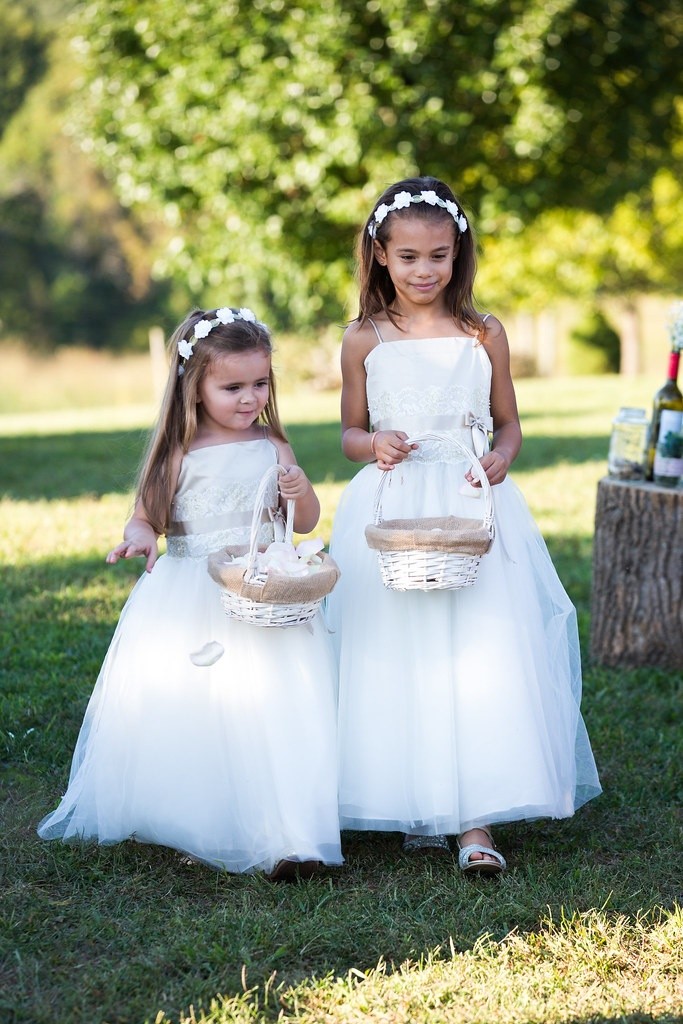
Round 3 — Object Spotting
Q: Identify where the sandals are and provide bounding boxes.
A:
[403,832,451,857]
[455,827,505,873]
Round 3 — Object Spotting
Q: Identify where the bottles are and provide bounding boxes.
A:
[647,346,683,487]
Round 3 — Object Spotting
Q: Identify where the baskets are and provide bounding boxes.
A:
[207,463,342,628]
[365,433,496,591]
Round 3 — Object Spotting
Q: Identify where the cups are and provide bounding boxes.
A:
[607,407,649,481]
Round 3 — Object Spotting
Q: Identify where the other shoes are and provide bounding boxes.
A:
[177,851,195,865]
[267,859,318,882]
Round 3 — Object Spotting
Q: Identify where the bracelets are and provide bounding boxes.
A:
[371,431,380,456]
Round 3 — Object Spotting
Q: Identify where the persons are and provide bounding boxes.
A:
[324,179,603,874]
[37,309,344,875]
